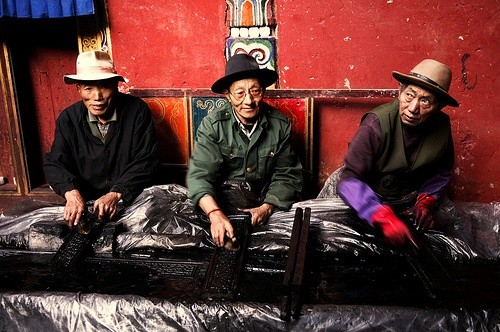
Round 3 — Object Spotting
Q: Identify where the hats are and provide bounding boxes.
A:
[391,59,460,106]
[63,50,128,84]
[211,54,278,94]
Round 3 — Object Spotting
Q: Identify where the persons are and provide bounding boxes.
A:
[336,58,460,253]
[43,51,158,228]
[184,54,306,248]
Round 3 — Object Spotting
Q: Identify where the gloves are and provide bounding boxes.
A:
[336,177,419,251]
[399,176,449,232]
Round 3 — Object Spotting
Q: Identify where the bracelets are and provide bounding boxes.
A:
[207,208,221,216]
